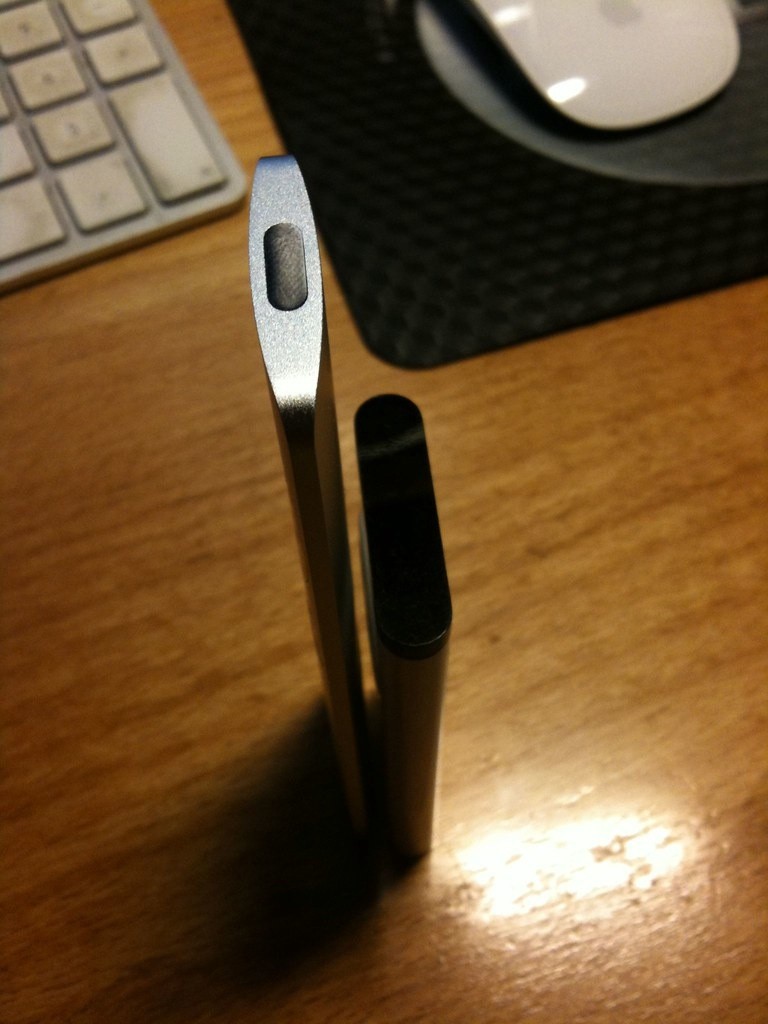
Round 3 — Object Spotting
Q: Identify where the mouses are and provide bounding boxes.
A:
[453,0,739,129]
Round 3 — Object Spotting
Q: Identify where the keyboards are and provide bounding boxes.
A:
[0,1,249,295]
